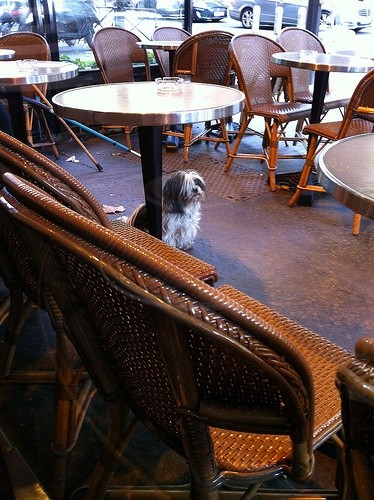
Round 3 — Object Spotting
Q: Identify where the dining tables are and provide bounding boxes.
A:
[52,82,247,242]
[0,48,77,158]
[314,133,374,221]
[273,51,374,157]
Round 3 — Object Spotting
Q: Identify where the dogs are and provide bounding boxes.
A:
[117,169,207,251]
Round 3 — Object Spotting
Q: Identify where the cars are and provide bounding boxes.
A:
[154,1,228,23]
[0,1,102,48]
[228,0,374,32]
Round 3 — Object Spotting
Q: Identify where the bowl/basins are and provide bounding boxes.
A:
[299,50,318,61]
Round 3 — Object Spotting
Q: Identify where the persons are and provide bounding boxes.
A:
[11,7,22,25]
[0,9,14,32]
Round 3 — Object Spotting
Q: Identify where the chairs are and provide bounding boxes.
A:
[0,27,374,235]
[0,130,374,500]
